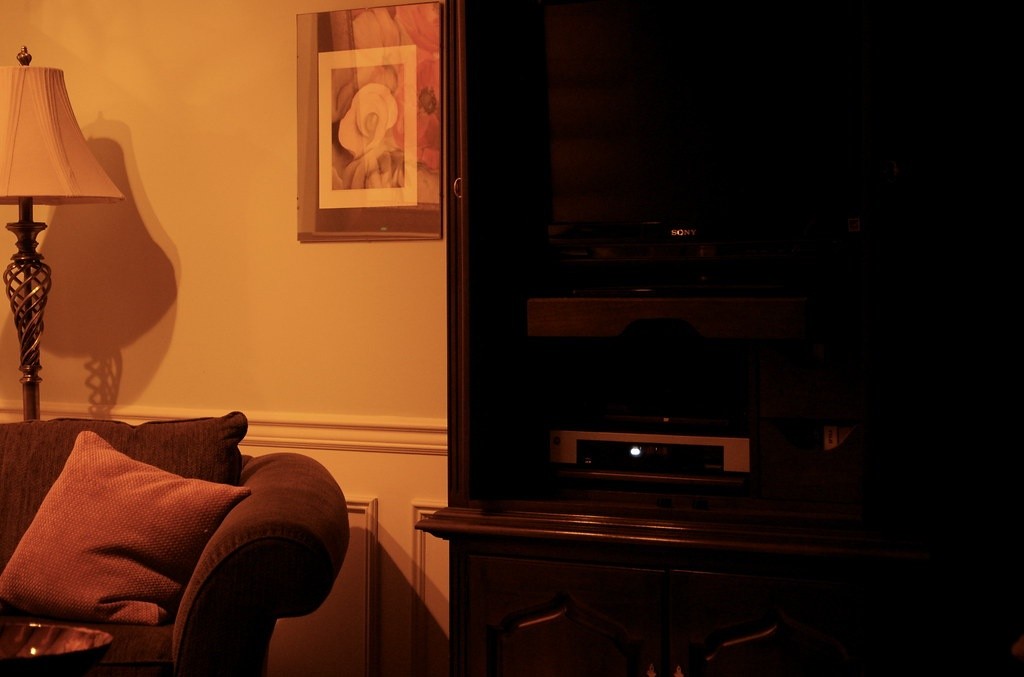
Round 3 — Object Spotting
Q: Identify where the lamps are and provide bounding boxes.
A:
[0,47,126,422]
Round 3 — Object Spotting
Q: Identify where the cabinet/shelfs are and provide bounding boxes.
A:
[763,363,861,499]
[467,553,870,677]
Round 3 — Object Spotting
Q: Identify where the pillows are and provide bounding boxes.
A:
[0,431,251,622]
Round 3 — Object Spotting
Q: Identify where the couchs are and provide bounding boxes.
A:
[0,411,351,677]
